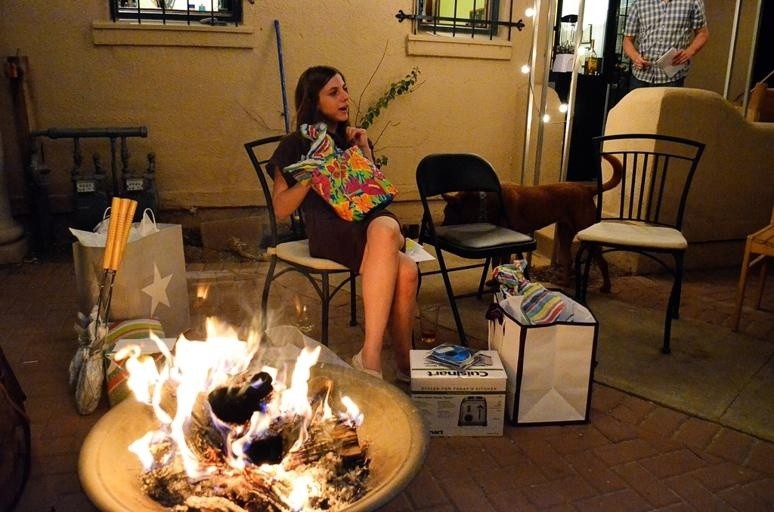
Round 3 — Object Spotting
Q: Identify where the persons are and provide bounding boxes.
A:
[619,1,710,96]
[262,64,419,387]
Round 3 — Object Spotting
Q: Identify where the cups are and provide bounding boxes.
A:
[418,302,440,343]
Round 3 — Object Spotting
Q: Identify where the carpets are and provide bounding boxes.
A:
[305,238,773,442]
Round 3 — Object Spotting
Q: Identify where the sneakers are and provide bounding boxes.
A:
[390,353,411,383]
[337,350,383,379]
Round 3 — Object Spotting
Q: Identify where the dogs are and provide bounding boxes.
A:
[440,152,623,291]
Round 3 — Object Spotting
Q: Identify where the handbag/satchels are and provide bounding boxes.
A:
[72,205,191,336]
[486,288,597,426]
[286,121,400,224]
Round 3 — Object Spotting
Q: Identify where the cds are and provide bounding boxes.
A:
[436,346,471,362]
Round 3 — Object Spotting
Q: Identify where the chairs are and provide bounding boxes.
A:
[415,152,536,346]
[242,133,357,346]
[572,133,707,355]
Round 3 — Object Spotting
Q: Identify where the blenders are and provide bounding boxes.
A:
[552,15,578,72]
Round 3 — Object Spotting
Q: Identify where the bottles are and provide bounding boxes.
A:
[585,39,599,76]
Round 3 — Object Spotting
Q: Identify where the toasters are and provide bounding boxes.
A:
[457,396,488,428]
